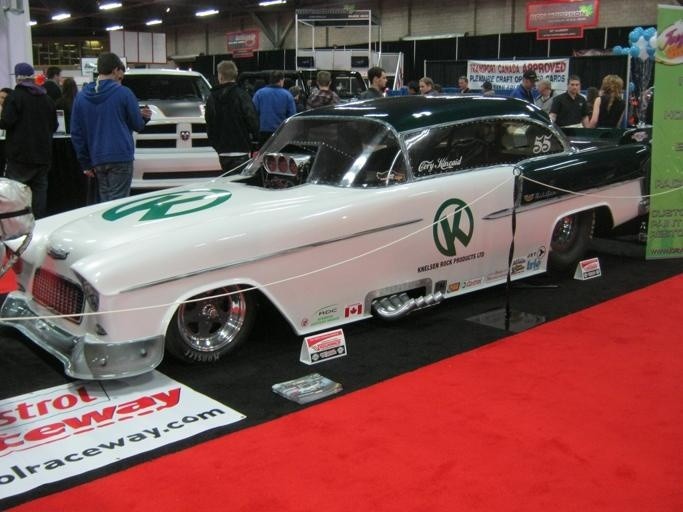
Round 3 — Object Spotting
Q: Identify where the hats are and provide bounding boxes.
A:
[8,63,34,75]
[481,82,492,90]
[524,70,539,82]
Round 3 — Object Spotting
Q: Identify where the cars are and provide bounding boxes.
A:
[232,69,366,102]
[117,68,250,191]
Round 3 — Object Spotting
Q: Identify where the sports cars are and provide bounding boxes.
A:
[0,92,652,381]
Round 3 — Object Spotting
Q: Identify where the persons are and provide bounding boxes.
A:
[536,78,559,112]
[252,73,297,148]
[408,77,443,95]
[549,75,590,128]
[286,70,339,112]
[360,67,388,100]
[510,70,539,104]
[589,75,627,129]
[482,81,496,97]
[459,77,472,92]
[0,52,152,220]
[587,87,599,121]
[205,59,259,171]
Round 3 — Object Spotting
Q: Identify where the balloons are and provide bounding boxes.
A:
[612,27,657,63]
